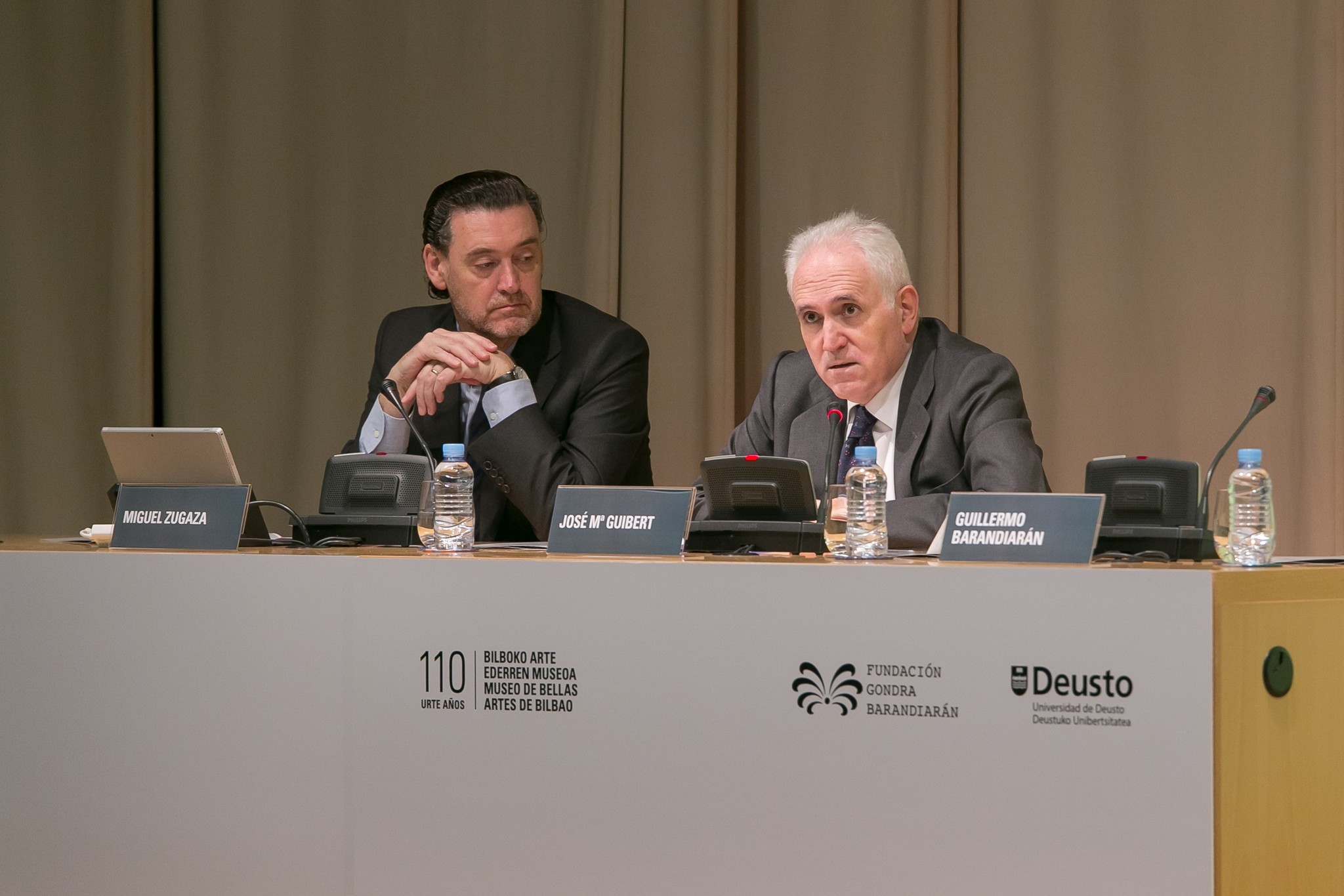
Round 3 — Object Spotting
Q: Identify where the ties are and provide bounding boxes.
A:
[466,382,491,542]
[835,405,877,496]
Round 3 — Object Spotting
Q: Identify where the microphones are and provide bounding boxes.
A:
[1193,385,1277,530]
[817,401,844,523]
[379,379,436,481]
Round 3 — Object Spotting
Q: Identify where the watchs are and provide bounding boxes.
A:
[487,365,528,389]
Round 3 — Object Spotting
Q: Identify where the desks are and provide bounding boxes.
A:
[0,554,1344,896]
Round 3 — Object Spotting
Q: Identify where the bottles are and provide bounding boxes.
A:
[1227,449,1276,564]
[434,442,475,550]
[845,445,889,559]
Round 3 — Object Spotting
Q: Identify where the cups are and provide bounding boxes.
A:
[1210,489,1273,562]
[822,484,849,559]
[416,480,476,550]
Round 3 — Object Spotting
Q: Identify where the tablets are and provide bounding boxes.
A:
[100,427,242,484]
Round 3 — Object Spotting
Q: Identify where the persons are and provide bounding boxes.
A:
[351,171,655,542]
[692,209,1051,551]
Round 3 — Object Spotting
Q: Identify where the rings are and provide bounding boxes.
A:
[431,369,439,375]
